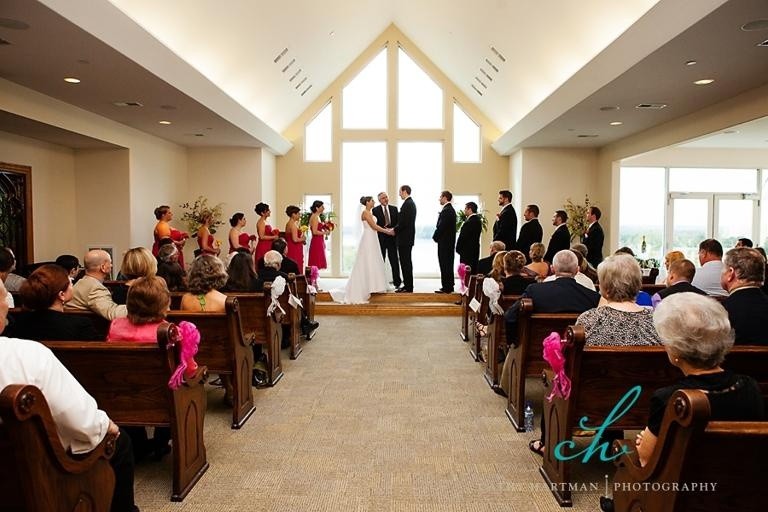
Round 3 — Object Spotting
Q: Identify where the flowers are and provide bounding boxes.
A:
[249,234,256,249]
[322,221,335,241]
[179,232,189,248]
[299,225,309,245]
[179,193,226,239]
[214,239,222,258]
[271,229,279,237]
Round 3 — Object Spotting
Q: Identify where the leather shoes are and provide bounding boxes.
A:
[395,288,407,292]
[435,289,444,292]
[454,301,462,304]
[529,440,545,457]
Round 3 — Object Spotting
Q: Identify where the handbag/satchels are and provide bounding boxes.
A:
[252,353,269,386]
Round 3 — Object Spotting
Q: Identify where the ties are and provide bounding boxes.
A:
[384,206,389,225]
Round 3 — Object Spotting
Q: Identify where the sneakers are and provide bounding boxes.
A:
[600,496,615,512]
[302,321,319,335]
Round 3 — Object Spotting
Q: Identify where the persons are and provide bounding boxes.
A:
[492,190,517,243]
[581,207,604,269]
[372,192,402,292]
[473,239,768,512]
[543,210,570,264]
[198,209,223,259]
[254,202,280,264]
[359,195,390,294]
[387,185,417,292]
[454,201,482,306]
[432,190,455,293]
[150,205,190,274]
[228,212,255,256]
[518,205,543,250]
[0,236,319,512]
[286,205,309,275]
[309,201,331,292]
